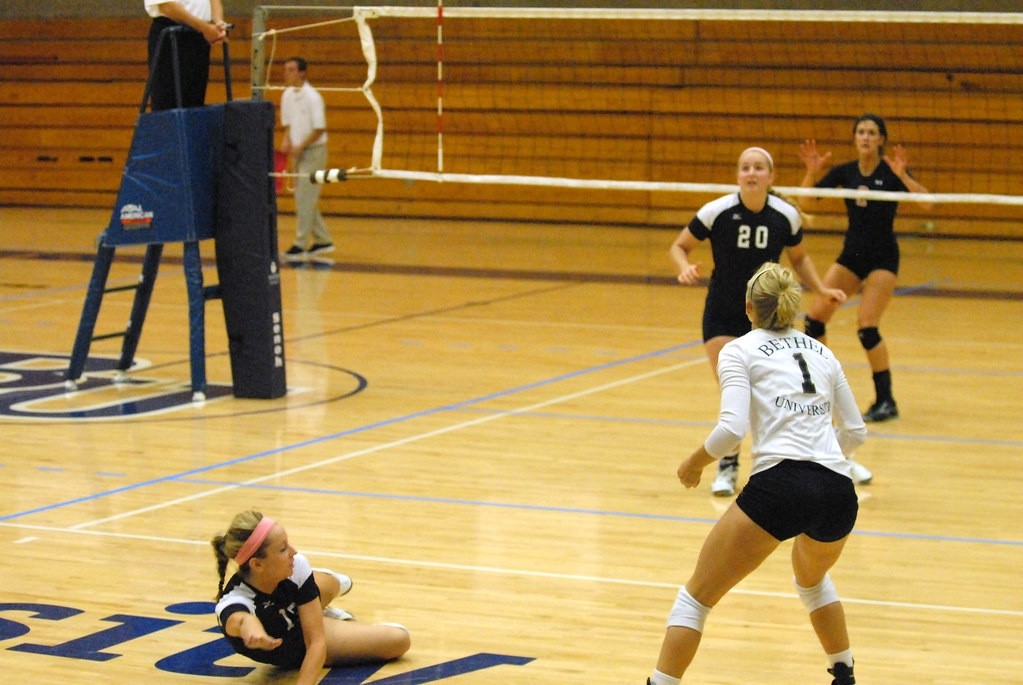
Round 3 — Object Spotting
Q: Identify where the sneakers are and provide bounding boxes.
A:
[323,605,356,620]
[311,567,353,598]
[861,400,900,423]
[846,459,872,484]
[711,467,739,495]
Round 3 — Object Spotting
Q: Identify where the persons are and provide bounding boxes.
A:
[795,112,934,424]
[670,146,875,497]
[143,0,226,112]
[277,58,338,259]
[639,263,869,685]
[209,511,411,685]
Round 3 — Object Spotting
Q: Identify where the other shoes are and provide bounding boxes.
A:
[309,244,335,256]
[283,245,308,260]
